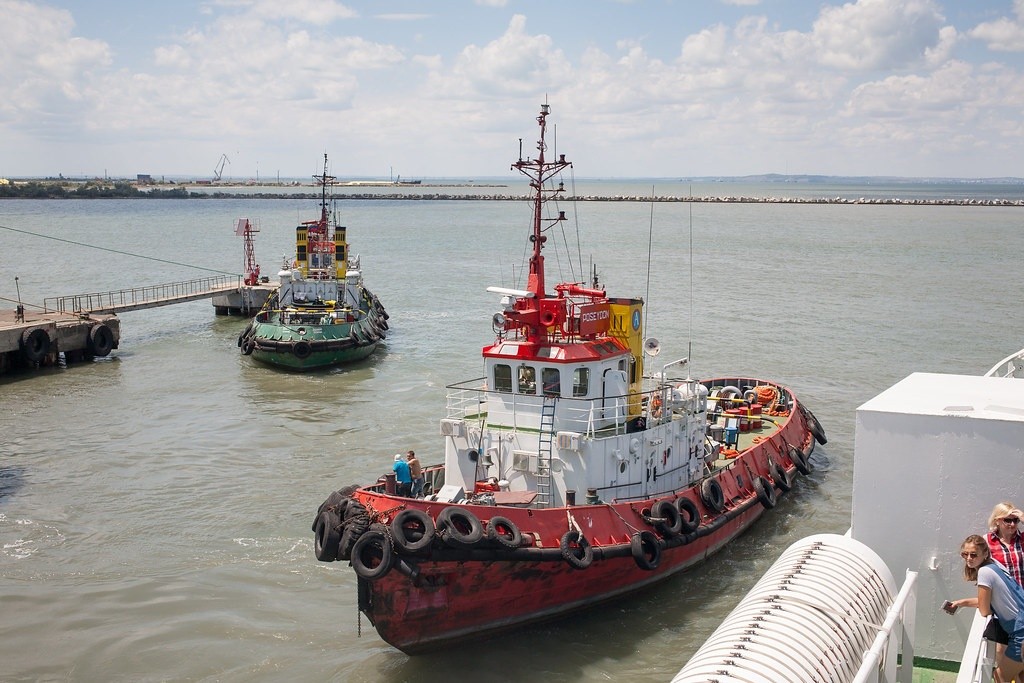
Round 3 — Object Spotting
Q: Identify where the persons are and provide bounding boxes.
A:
[946,534,1024,683]
[255,265,260,279]
[981,501,1024,683]
[393,451,425,499]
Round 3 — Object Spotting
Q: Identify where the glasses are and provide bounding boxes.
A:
[1003,518,1020,524]
[961,552,981,559]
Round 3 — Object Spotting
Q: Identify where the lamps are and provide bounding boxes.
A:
[492,313,508,344]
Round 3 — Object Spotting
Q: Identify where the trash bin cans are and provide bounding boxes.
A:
[725,427,737,444]
[710,424,724,441]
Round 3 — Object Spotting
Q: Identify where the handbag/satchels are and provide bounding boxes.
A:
[983,614,1010,645]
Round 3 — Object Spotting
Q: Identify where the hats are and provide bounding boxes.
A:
[394,454,403,462]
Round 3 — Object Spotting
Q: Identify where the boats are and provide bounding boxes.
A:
[249,151,382,372]
[311,87,815,655]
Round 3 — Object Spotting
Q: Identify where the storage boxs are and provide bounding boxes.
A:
[309,268,327,279]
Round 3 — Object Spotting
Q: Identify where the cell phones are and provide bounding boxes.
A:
[941,600,959,615]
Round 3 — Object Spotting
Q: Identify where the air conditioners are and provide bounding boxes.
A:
[557,432,583,453]
[439,419,465,437]
[512,450,542,473]
